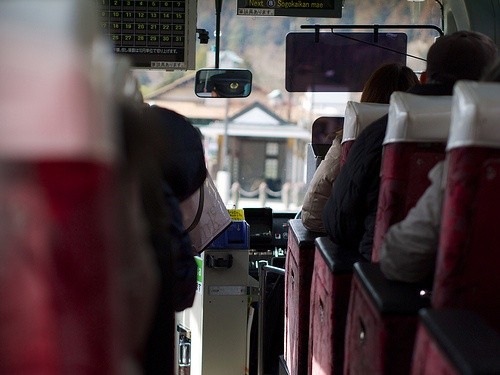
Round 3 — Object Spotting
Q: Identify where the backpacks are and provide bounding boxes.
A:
[127,102,209,314]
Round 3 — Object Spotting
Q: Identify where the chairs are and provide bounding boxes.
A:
[255,78,500,375]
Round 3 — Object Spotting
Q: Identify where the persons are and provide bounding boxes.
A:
[209,75,246,97]
[116,29,500,375]
